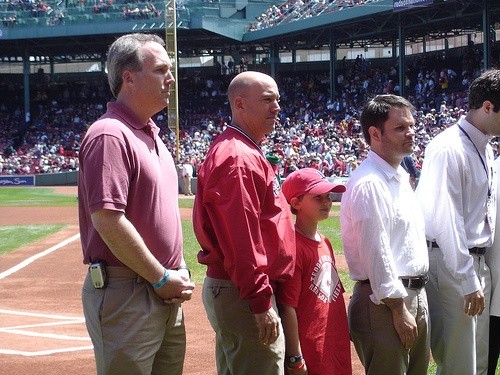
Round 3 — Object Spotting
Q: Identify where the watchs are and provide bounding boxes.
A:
[287,355,302,362]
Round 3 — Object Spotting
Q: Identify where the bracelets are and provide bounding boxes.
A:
[287,360,305,371]
[152,270,169,288]
[178,266,191,277]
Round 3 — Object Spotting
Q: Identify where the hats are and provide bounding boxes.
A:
[282,168,346,204]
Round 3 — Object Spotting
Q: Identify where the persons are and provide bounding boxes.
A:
[341,94,431,375]
[193,71,296,375]
[415,70,500,375]
[228,58,233,73]
[278,168,352,375]
[183,159,194,196]
[0,54,498,184]
[247,0,374,32]
[485,156,500,375]
[78,34,196,375]
[0,0,162,29]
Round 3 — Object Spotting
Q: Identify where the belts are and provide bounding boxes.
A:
[358,276,425,289]
[427,240,486,255]
